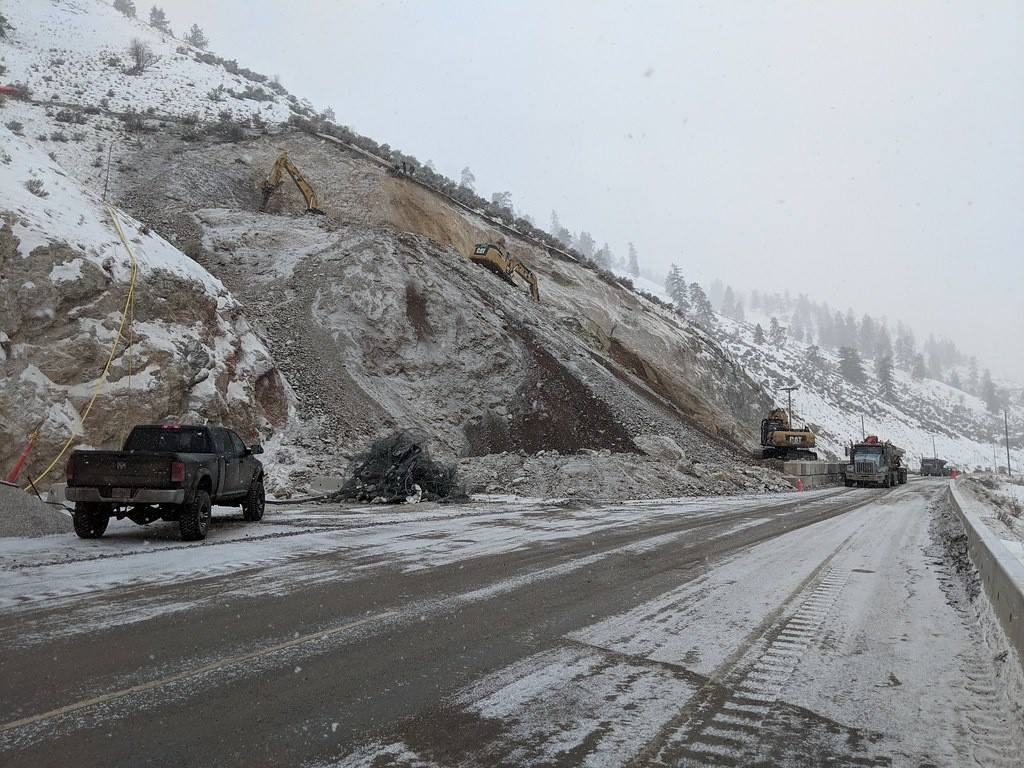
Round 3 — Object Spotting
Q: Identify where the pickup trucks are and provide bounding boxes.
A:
[65,418,268,540]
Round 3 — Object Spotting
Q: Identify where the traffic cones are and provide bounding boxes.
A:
[951,468,956,479]
[797,478,803,491]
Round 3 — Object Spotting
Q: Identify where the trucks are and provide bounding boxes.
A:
[845,438,907,488]
[920,453,948,476]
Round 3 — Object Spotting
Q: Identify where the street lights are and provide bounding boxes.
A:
[780,387,798,427]
[928,434,937,458]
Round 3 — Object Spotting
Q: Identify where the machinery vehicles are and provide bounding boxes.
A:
[468,237,540,302]
[751,407,818,461]
[261,152,324,214]
